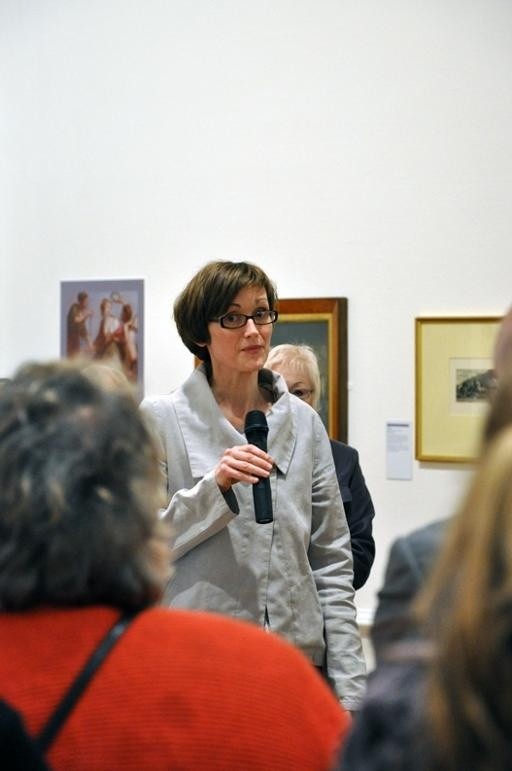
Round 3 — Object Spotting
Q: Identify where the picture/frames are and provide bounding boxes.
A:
[413,315,508,464]
[193,296,350,451]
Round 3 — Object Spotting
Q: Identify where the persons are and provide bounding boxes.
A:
[0,359,358,769]
[144,253,366,716]
[62,289,139,387]
[262,341,378,591]
[367,305,511,660]
[339,426,509,770]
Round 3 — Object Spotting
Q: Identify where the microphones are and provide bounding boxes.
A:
[244,410,273,524]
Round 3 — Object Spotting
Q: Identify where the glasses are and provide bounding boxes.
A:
[210,310,278,328]
